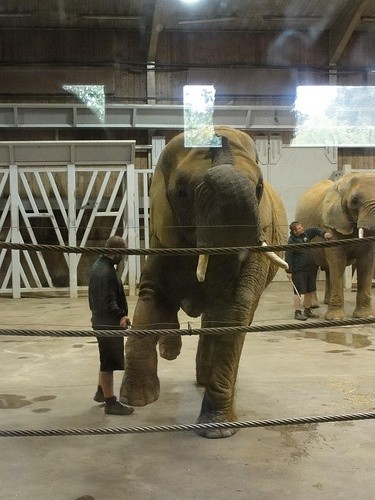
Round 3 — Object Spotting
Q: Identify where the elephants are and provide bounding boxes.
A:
[295,169,375,323]
[119,125,290,439]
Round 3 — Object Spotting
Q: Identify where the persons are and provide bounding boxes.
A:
[285,222,332,320]
[87,235,134,416]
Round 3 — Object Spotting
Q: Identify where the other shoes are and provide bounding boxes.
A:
[104,401,134,415]
[94,391,117,402]
[294,311,307,320]
[303,311,319,318]
